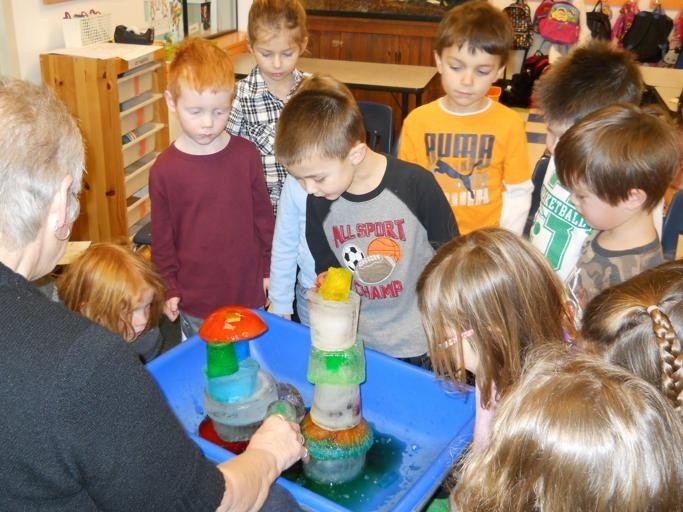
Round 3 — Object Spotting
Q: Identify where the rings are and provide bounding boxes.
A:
[302,448,308,458]
[297,432,305,445]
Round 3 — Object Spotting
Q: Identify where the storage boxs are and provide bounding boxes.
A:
[125,189,162,230]
[116,92,168,134]
[117,64,165,103]
[122,152,180,197]
[121,123,171,167]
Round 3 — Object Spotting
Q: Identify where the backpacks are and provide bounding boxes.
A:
[623,4,673,63]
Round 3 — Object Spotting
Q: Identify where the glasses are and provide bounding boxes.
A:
[434,326,499,352]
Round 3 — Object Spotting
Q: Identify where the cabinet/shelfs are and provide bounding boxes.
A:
[39,35,169,248]
[302,16,470,146]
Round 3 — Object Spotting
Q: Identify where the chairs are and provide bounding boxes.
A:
[343,101,396,156]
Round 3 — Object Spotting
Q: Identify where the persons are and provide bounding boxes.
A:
[416,228,578,453]
[521,41,643,289]
[395,0,535,236]
[583,259,683,410]
[268,73,362,321]
[446,346,683,512]
[224,0,314,217]
[275,89,461,373]
[0,78,309,512]
[554,104,680,334]
[148,37,275,339]
[40,241,163,363]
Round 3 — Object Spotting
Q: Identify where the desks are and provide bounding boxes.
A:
[216,48,440,120]
